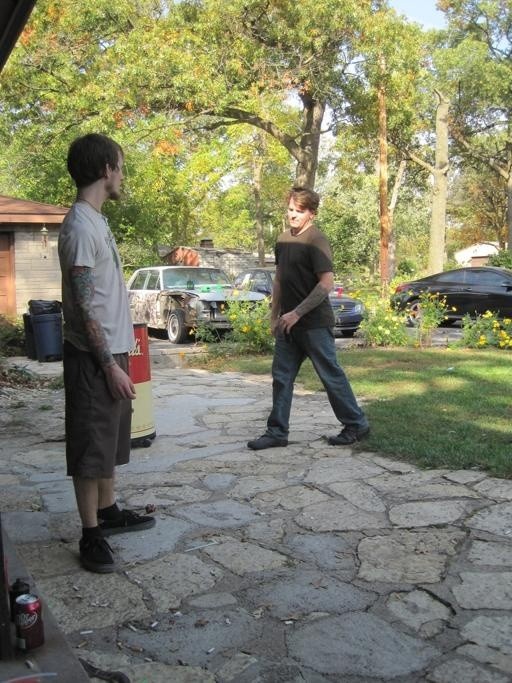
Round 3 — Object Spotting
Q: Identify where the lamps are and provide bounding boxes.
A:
[40,224,49,249]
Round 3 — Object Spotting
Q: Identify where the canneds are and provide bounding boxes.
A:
[13,593,44,652]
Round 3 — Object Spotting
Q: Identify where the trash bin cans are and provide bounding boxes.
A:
[23,312,63,363]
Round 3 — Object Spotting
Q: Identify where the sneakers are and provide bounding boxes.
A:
[328,427,369,445]
[79,539,114,573]
[98,510,155,537]
[248,435,288,449]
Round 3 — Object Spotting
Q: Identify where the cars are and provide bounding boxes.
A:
[125,265,267,345]
[234,269,365,337]
[390,267,512,327]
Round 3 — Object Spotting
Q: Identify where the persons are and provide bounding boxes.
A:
[62,132,156,571]
[246,185,371,450]
